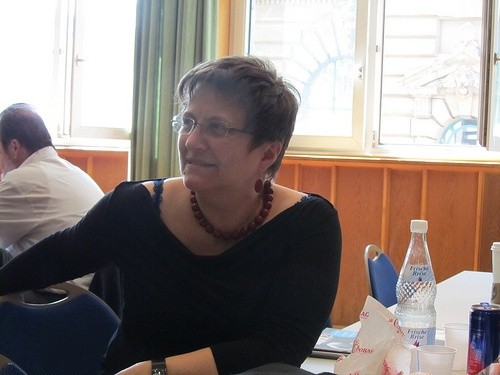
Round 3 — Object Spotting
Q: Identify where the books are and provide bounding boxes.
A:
[311,328,360,359]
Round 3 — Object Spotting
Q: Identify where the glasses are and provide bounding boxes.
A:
[168,114,258,140]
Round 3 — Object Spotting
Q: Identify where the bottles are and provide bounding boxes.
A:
[392,220,437,347]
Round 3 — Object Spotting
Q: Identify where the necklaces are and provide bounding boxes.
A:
[190,180,274,241]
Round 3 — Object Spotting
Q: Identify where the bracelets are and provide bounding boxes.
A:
[152,357,167,375]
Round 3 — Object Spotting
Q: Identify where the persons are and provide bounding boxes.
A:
[0,56,341,375]
[0,104,104,304]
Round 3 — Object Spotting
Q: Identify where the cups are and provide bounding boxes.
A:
[443,322,469,370]
[416,345,457,375]
[490,241,500,305]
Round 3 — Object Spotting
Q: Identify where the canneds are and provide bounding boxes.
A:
[466,302,500,375]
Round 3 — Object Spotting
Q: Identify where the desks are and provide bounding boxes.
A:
[300,270,493,375]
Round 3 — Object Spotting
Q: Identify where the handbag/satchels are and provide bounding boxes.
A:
[334,295,421,375]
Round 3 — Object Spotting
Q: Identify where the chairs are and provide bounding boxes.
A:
[364,245,399,307]
[0,283,120,375]
